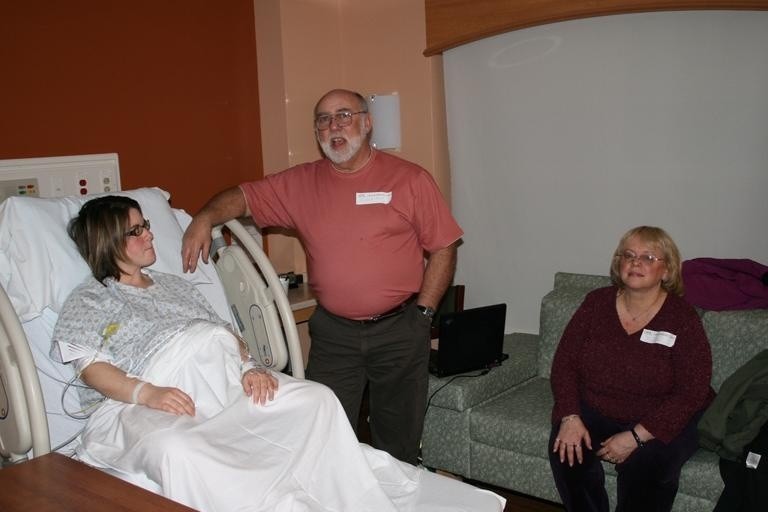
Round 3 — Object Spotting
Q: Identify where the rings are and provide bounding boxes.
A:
[574,443,581,448]
[607,454,611,459]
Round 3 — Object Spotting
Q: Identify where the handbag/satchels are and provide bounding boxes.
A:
[681,258,768,311]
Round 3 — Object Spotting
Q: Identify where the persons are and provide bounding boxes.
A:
[548,227,719,511]
[48,195,407,512]
[699,348,768,511]
[180,90,463,466]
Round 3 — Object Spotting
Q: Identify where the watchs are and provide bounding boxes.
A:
[415,304,434,326]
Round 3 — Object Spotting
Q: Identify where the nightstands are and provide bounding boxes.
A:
[288,281,318,376]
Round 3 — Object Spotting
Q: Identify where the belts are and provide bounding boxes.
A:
[317,293,417,324]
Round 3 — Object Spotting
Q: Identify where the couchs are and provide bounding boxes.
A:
[418,270,768,512]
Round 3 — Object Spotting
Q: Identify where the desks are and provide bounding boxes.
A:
[0,451,201,512]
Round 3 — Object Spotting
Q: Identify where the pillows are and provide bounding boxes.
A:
[697,309,768,393]
[537,289,596,380]
[0,186,211,324]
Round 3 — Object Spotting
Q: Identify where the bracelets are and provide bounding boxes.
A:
[631,427,646,450]
[559,415,579,421]
[133,380,149,405]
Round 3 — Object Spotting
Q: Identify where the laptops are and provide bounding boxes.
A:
[428,302,507,379]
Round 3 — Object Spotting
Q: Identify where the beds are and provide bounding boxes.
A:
[0,191,508,512]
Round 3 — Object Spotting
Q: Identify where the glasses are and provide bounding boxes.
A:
[314,111,368,131]
[123,219,150,236]
[616,252,668,266]
